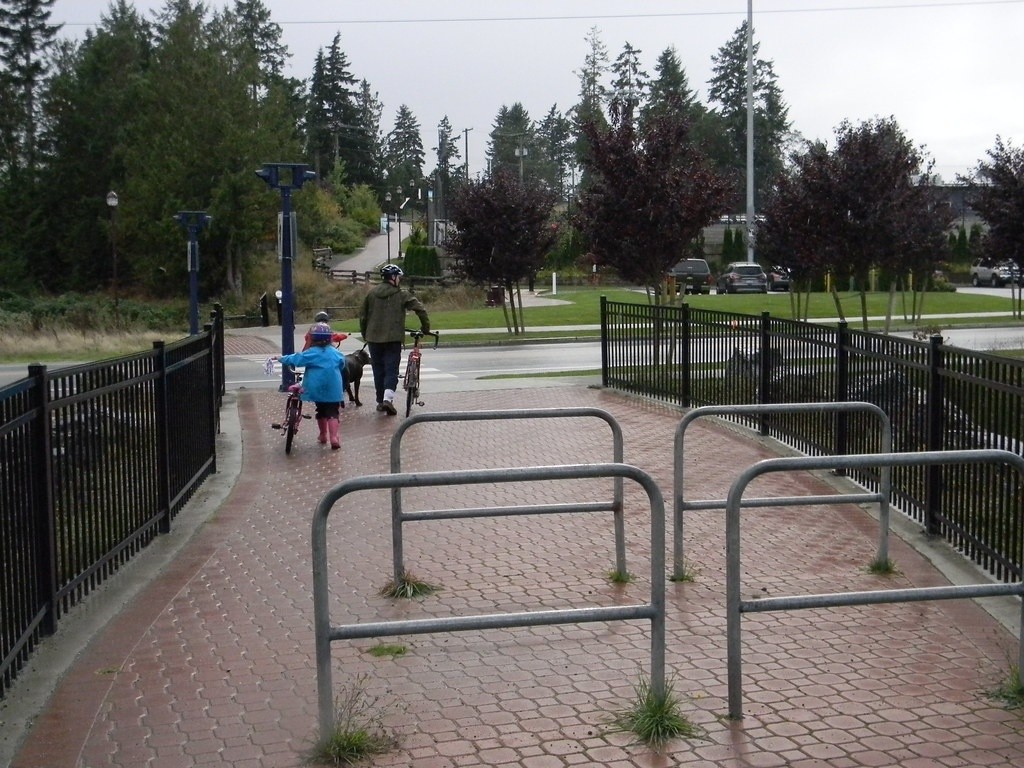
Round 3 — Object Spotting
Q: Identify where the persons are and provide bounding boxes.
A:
[302,311,348,352]
[359,264,430,416]
[274,322,345,450]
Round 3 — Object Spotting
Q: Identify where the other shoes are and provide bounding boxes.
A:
[377,403,384,411]
[383,400,397,415]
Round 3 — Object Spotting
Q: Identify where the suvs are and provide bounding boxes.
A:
[969,257,1020,288]
[715,261,768,294]
[655,258,711,295]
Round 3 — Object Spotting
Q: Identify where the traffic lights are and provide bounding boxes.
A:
[172,211,211,230]
[254,161,317,192]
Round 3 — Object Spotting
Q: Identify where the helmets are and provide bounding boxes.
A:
[380,264,404,276]
[315,311,329,321]
[308,322,332,335]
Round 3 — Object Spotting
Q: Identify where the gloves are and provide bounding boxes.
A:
[421,326,429,336]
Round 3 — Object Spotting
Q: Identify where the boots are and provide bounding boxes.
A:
[328,419,341,449]
[317,418,328,443]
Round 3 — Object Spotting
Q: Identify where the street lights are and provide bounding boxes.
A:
[513,147,528,191]
[396,186,404,260]
[410,178,415,234]
[106,189,119,333]
[384,192,391,265]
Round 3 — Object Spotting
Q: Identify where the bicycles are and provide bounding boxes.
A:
[268,356,312,454]
[398,327,439,418]
[336,332,351,349]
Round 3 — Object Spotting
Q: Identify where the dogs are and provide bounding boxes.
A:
[340,350,372,408]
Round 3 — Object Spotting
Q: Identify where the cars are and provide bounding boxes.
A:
[769,265,794,291]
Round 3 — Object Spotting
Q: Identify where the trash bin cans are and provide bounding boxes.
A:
[491,286,505,306]
